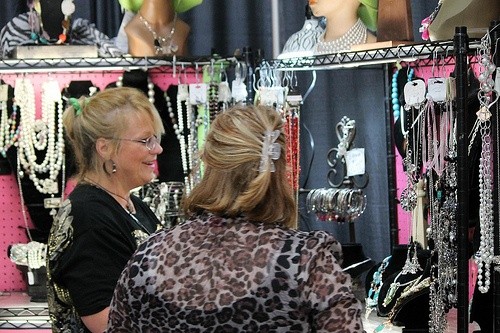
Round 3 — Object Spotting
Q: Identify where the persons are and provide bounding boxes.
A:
[281,0,378,56]
[126,0,192,59]
[46,87,163,333]
[106,104,365,333]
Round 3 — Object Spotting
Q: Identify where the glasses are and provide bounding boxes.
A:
[102,136,161,150]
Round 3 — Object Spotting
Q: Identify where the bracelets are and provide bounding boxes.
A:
[26,241,44,270]
[306,188,367,226]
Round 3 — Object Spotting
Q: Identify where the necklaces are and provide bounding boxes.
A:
[137,9,180,58]
[116,59,300,216]
[81,173,134,213]
[0,74,68,196]
[316,18,368,55]
[26,0,76,46]
[342,29,496,333]
